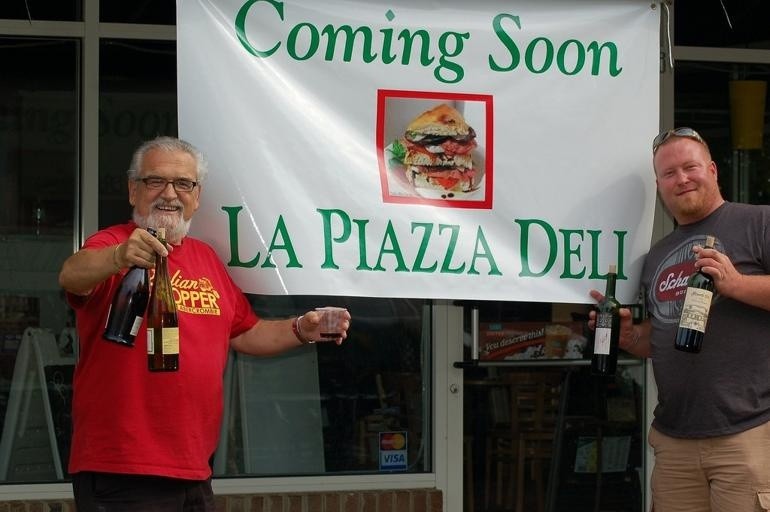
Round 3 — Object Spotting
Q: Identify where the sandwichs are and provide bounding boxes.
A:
[401,102,477,192]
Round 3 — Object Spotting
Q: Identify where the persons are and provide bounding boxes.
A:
[584,126,770,512]
[54,135,353,511]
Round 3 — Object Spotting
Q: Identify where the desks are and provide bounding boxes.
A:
[396,373,508,428]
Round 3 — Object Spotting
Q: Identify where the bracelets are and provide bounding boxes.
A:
[293,315,316,349]
[112,244,126,269]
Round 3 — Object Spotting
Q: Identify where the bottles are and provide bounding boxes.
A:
[592,262,624,379]
[100,228,161,349]
[672,232,723,352]
[145,227,181,373]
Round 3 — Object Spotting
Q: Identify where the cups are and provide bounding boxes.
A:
[315,306,348,340]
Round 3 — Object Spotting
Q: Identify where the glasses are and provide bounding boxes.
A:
[133,177,201,191]
[652,128,706,156]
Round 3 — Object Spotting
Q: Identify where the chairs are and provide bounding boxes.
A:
[373,374,475,512]
[481,372,564,512]
